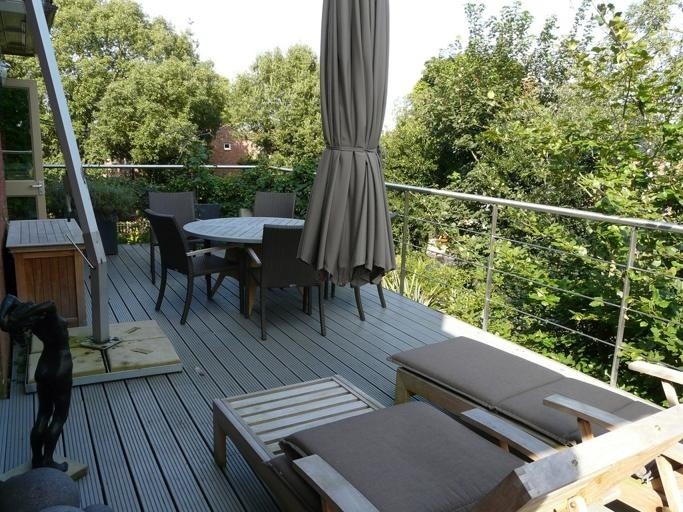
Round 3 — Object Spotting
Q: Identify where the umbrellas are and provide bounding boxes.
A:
[293,0,400,296]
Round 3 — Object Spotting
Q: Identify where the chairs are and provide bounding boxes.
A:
[212,374,682,510]
[387,337,683,512]
[142,190,386,341]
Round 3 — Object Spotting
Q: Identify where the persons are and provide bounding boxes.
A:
[0,292,74,473]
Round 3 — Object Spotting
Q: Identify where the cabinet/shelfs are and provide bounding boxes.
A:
[6,217,89,327]
